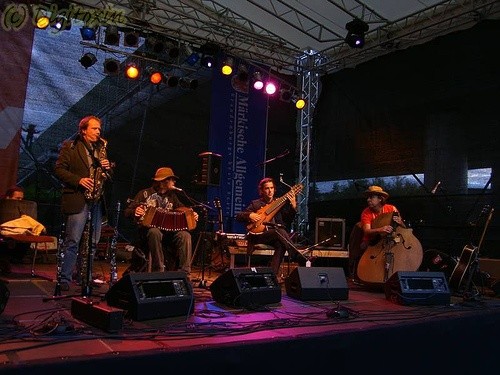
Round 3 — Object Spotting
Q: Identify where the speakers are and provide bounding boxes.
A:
[194,152,222,187]
[209,267,282,309]
[385,271,451,306]
[105,270,195,321]
[284,267,349,302]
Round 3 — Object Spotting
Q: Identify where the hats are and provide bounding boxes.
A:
[153,167,180,182]
[361,185,389,203]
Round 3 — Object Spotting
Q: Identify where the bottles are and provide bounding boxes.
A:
[112,267,117,285]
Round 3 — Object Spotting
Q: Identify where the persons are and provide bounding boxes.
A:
[54,115,109,293]
[236,177,310,285]
[124,167,199,286]
[349,185,407,284]
[0,185,31,265]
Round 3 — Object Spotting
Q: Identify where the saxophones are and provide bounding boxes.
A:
[84,137,109,201]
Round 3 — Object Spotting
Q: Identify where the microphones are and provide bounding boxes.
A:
[262,221,284,227]
[431,181,441,194]
[70,130,81,149]
[171,186,183,192]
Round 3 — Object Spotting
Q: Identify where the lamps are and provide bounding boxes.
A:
[345,19,369,49]
[219,56,308,111]
[122,61,140,82]
[54,18,70,32]
[79,53,98,67]
[161,40,180,60]
[144,65,164,86]
[103,59,122,78]
[103,24,120,46]
[30,9,50,30]
[144,37,166,54]
[121,30,140,47]
[182,44,199,66]
[199,41,221,70]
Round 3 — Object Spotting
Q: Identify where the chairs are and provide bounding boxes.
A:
[248,210,292,274]
[0,206,58,279]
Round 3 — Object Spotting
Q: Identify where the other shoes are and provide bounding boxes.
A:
[276,276,285,284]
[90,279,104,287]
[292,252,308,267]
[60,282,70,291]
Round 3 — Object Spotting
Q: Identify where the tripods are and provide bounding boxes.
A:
[42,137,104,301]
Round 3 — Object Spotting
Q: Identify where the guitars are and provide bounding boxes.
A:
[245,183,304,235]
[448,204,494,293]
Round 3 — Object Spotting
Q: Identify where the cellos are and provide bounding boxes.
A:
[356,212,424,286]
